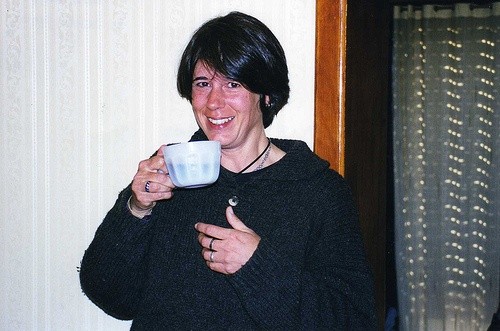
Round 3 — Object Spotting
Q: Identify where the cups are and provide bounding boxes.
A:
[157,141,221,188]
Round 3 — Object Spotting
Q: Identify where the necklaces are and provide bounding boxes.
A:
[220,137,272,206]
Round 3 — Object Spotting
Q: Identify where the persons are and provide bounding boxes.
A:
[79,11,379,331]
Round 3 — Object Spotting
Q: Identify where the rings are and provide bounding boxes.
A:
[210,251,214,262]
[146,181,150,192]
[210,238,215,250]
